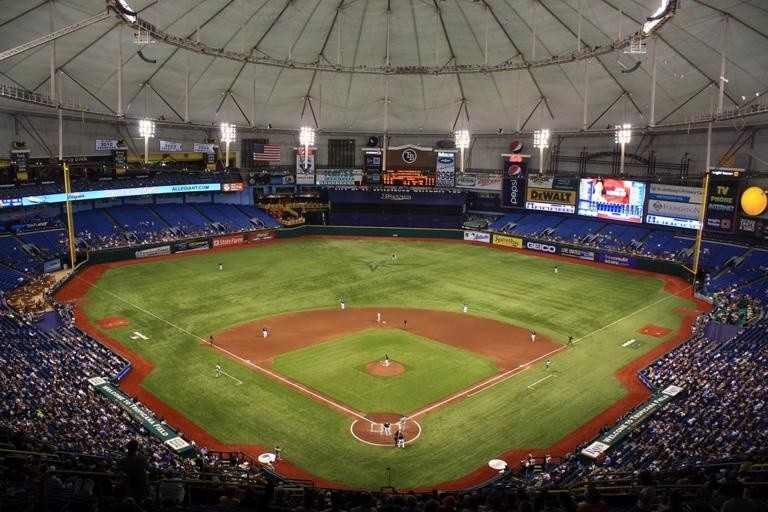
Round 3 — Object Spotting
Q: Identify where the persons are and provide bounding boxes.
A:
[339,251,469,328]
[366,329,768,511]
[0,208,280,279]
[477,214,766,334]
[0,277,331,512]
